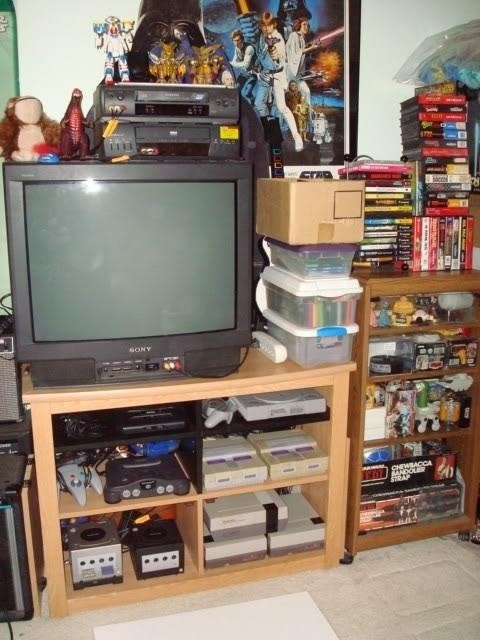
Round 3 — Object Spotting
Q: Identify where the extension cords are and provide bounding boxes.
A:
[251,330,288,364]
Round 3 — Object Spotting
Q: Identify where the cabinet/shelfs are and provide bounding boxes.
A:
[21,346,357,617]
[346,269,480,557]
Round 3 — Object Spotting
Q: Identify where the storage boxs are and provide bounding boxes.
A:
[255,179,366,371]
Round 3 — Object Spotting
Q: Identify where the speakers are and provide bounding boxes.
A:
[130,518,186,580]
[0,334,26,424]
[67,518,123,591]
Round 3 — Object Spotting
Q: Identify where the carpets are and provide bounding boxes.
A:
[90,590,341,640]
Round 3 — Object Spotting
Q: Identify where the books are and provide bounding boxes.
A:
[332,79,476,274]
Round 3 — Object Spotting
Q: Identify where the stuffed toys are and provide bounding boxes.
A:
[0,94,61,167]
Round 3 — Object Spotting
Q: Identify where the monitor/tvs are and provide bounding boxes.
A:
[2,158,255,389]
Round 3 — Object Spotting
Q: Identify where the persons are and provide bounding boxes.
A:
[285,79,311,144]
[286,8,312,89]
[248,9,304,153]
[227,29,264,106]
